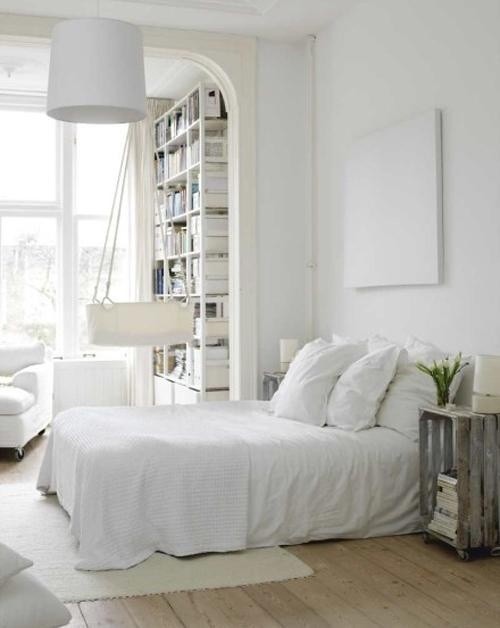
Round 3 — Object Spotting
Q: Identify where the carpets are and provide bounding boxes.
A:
[2,427,315,604]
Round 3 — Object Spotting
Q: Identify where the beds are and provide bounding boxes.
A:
[51,361,470,558]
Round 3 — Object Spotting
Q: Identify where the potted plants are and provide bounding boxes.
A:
[417,357,469,408]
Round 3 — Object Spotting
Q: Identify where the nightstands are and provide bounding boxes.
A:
[262,371,290,401]
[419,400,500,561]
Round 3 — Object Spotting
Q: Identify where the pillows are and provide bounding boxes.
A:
[267,337,357,427]
[375,366,465,442]
[326,346,404,431]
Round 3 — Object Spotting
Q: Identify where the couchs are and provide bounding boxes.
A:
[1,335,84,461]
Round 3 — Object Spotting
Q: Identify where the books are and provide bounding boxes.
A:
[426,471,460,542]
[154,87,227,392]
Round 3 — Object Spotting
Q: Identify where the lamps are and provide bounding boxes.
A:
[472,354,500,414]
[47,0,147,124]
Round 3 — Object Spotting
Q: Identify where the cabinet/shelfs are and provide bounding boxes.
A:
[152,82,229,392]
